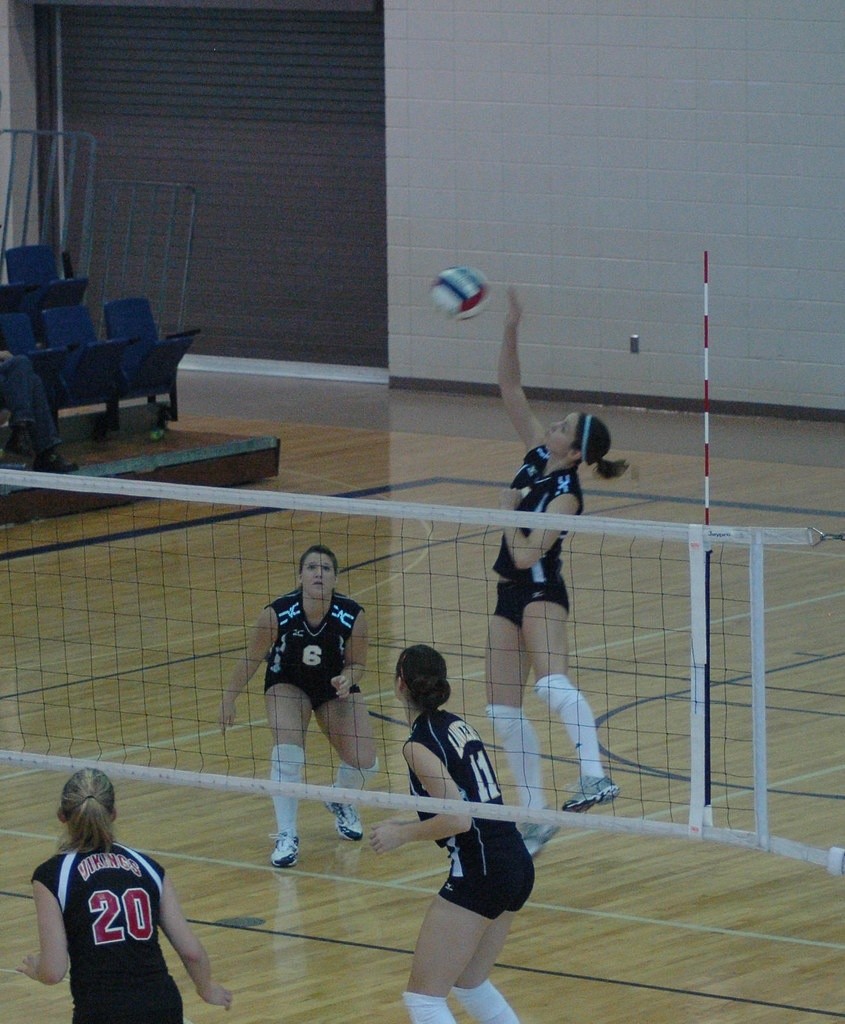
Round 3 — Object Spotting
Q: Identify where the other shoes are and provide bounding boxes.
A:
[3,422,36,456]
[31,451,78,473]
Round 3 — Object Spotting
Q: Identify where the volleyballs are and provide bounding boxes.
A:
[434,268,492,318]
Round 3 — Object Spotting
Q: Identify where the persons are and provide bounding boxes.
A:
[16,767,233,1024]
[215,544,378,868]
[483,284,631,857]
[369,644,535,1024]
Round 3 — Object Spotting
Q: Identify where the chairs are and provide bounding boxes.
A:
[0,245,200,457]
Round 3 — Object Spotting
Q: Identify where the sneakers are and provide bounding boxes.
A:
[325,786,363,841]
[563,775,620,812]
[268,830,298,867]
[518,820,559,856]
